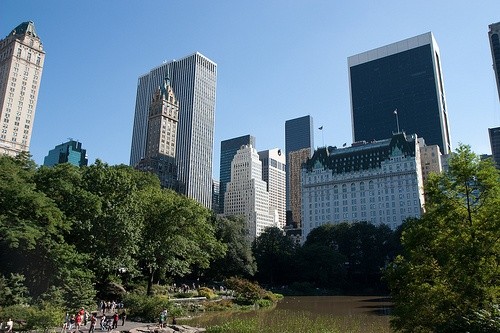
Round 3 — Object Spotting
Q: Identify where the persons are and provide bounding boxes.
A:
[6,317,13,333]
[61,322,68,333]
[121,311,126,326]
[89,315,97,333]
[68,299,127,333]
[112,312,119,329]
[0,320,5,333]
[159,309,168,328]
[174,281,227,295]
